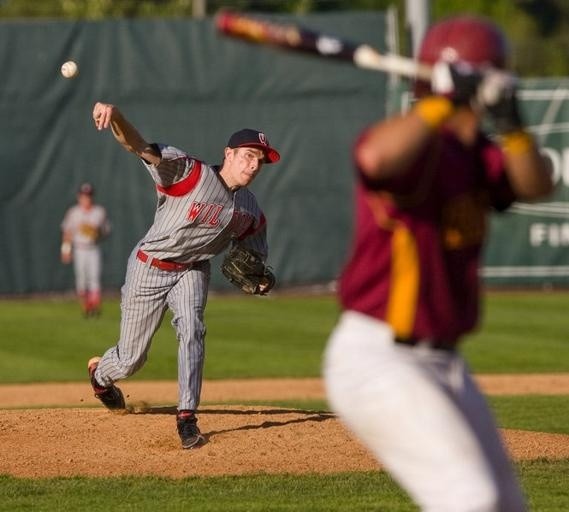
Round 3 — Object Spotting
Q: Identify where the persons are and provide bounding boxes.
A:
[58,183,113,317]
[87,98,281,449]
[323,19,553,511]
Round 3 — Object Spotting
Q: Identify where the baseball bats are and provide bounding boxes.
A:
[214,8,515,107]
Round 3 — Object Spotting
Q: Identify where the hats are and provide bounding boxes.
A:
[227,128,280,163]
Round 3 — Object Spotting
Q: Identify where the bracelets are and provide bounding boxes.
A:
[502,131,540,157]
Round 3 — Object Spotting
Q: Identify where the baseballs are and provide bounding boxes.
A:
[61,60,77,78]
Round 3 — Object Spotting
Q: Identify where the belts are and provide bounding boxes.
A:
[137,250,208,271]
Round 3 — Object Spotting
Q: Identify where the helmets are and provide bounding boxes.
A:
[413,16,505,97]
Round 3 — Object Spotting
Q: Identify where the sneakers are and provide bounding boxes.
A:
[88,356,125,410]
[176,408,205,449]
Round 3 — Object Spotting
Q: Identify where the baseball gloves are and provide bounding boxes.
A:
[220,245,274,296]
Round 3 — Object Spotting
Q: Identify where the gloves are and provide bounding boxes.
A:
[431,58,522,143]
